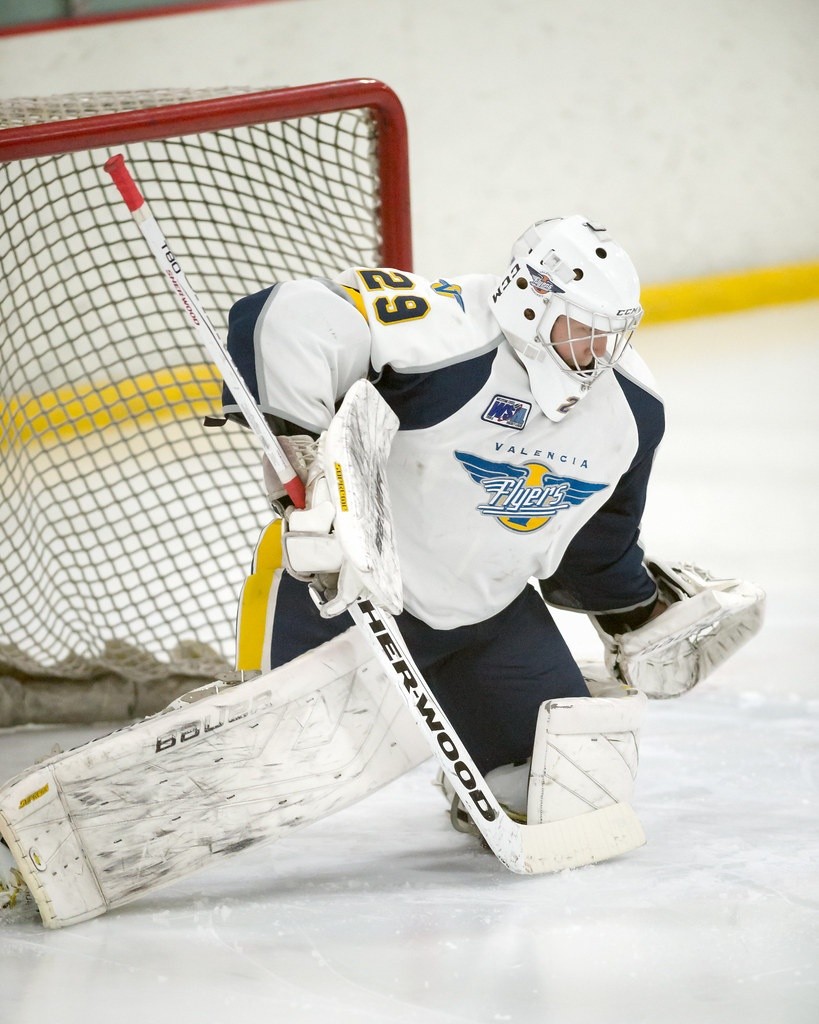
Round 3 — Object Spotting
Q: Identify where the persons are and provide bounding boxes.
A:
[0,216,766,931]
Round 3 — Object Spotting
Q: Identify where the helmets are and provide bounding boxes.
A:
[490,215,642,423]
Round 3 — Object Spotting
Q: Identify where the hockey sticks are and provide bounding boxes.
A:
[104,154,644,874]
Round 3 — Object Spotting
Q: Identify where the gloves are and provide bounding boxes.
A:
[262,431,340,601]
